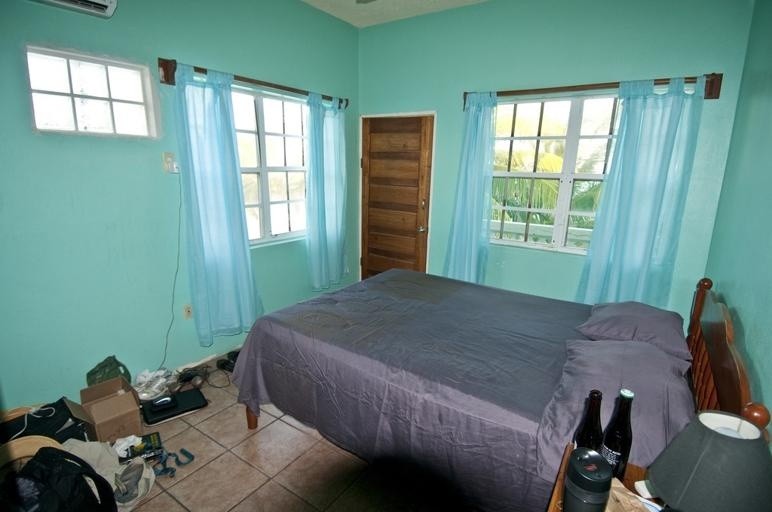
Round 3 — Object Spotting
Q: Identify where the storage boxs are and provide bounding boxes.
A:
[80,374,142,443]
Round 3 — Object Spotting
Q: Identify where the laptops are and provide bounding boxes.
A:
[142,387,207,425]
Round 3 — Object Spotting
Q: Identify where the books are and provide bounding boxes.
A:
[110,432,164,466]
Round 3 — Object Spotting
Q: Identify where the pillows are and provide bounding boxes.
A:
[574,300,693,362]
[535,336,695,465]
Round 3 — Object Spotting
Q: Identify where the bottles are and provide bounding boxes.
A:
[599,390,634,482]
[573,390,603,450]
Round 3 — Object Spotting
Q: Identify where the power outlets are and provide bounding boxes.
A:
[159,152,178,173]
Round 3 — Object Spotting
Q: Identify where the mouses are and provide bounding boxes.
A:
[151,395,172,407]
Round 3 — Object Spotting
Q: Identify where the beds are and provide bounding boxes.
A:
[232,268,772,511]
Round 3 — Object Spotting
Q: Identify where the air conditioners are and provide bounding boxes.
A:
[34,0,118,19]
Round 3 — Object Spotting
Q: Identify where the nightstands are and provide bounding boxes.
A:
[542,440,671,511]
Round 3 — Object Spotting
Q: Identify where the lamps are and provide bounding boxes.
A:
[643,408,772,509]
